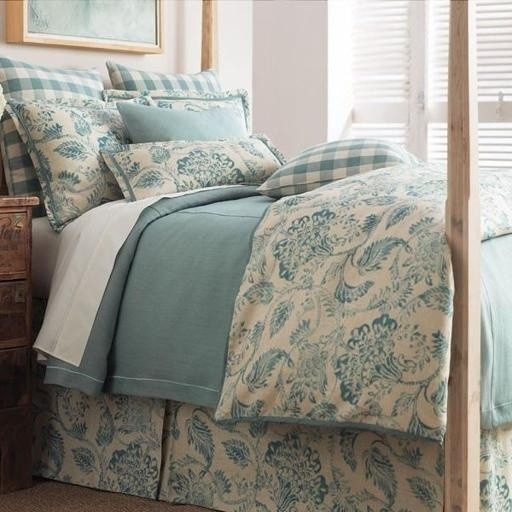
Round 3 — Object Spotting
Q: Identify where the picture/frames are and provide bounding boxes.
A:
[7,1,165,55]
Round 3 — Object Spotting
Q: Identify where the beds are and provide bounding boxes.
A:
[32,186,512,512]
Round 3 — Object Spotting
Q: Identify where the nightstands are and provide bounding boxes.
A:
[0,197,40,493]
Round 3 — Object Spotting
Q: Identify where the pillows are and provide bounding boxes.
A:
[256,138,419,200]
[115,102,244,143]
[1,56,105,218]
[100,89,252,134]
[4,90,162,233]
[105,59,221,91]
[102,132,287,201]
